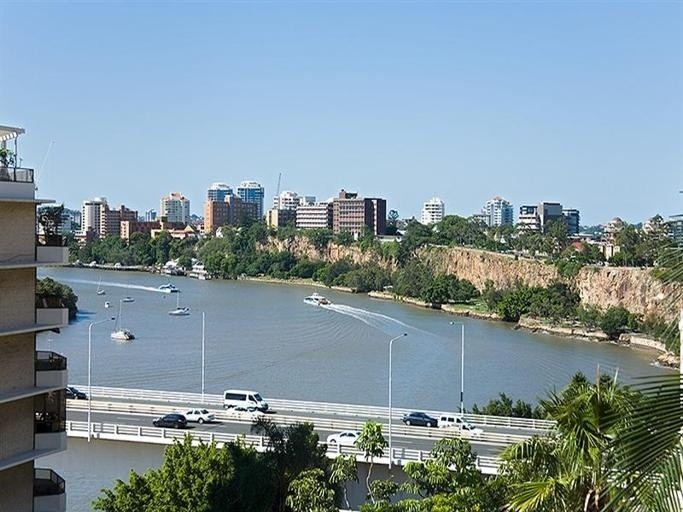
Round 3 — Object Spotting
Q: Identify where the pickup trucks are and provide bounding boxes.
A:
[441,420,485,437]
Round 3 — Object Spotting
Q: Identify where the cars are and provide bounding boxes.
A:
[400,410,438,427]
[184,408,214,424]
[151,413,187,429]
[66,386,87,401]
[326,429,366,447]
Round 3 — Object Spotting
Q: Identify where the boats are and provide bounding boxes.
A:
[167,288,191,316]
[303,292,333,307]
[110,299,135,341]
[95,289,134,309]
[158,282,180,293]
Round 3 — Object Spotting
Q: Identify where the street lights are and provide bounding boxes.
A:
[86,317,115,441]
[185,308,206,404]
[388,331,407,469]
[449,321,465,417]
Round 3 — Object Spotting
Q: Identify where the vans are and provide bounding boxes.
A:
[222,387,268,411]
[437,413,471,429]
